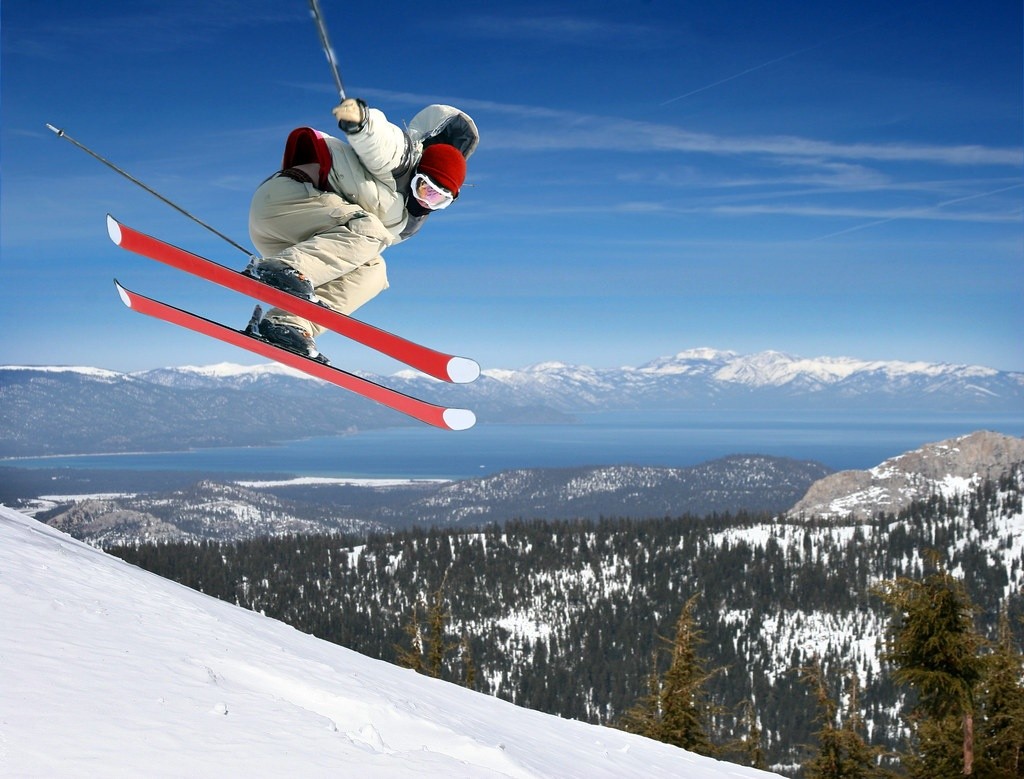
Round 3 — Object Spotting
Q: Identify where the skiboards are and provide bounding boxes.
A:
[105,212,482,432]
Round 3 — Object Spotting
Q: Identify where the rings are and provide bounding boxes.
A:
[344,105,348,112]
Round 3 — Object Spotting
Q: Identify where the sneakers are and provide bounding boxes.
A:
[259,319,331,365]
[255,257,317,298]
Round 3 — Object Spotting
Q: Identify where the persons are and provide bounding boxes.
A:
[242,97,480,366]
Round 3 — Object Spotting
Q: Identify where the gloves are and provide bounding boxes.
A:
[333,99,364,124]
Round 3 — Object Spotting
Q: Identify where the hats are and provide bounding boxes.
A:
[417,144,466,196]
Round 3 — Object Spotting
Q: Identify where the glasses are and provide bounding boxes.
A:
[410,174,453,209]
